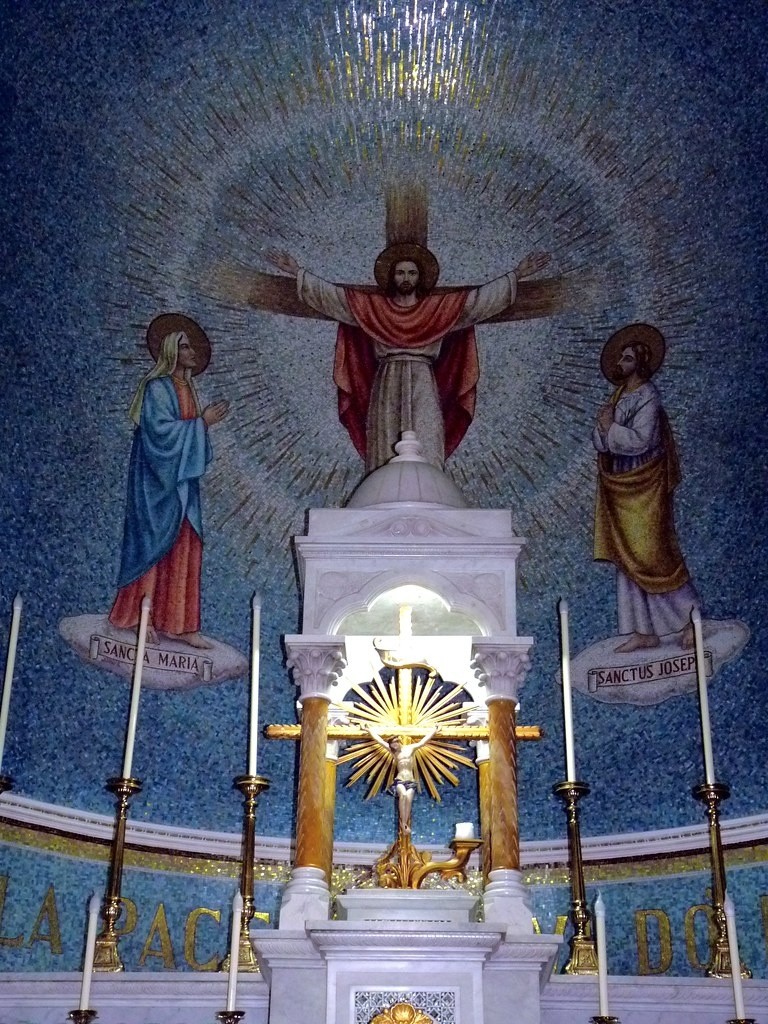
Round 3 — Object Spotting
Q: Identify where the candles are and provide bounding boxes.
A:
[122,591,151,778]
[0,593,21,775]
[249,595,262,777]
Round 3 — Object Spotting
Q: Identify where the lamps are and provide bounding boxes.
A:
[691,606,716,785]
[724,887,746,1019]
[226,889,244,1012]
[80,892,100,1010]
[595,889,610,1016]
[559,597,578,781]
